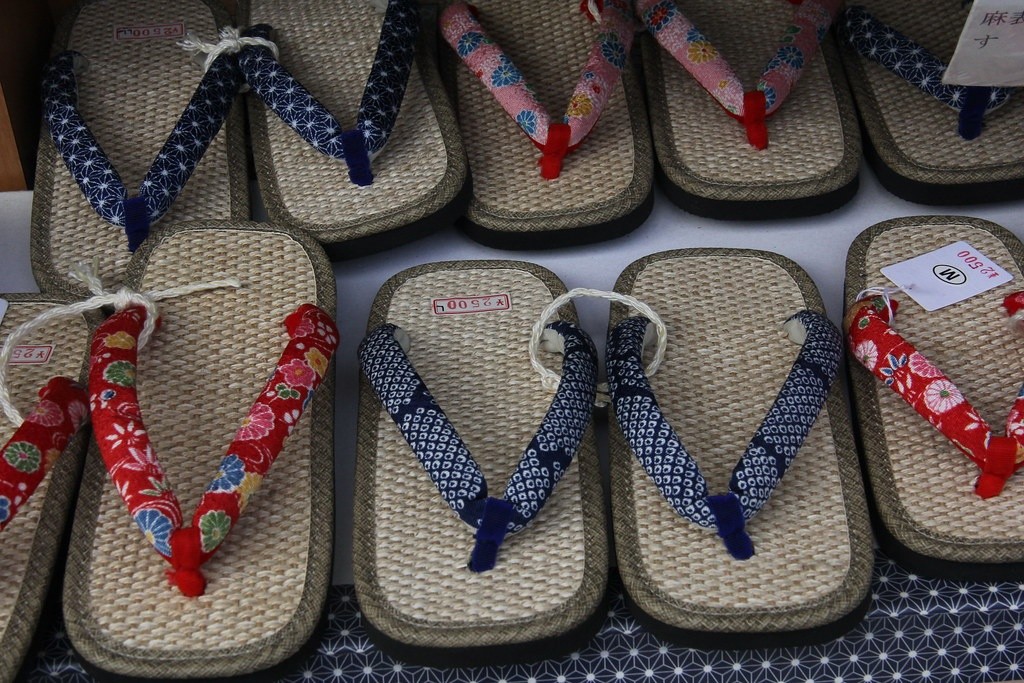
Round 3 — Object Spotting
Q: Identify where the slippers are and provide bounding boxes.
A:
[0,0,1024,678]
